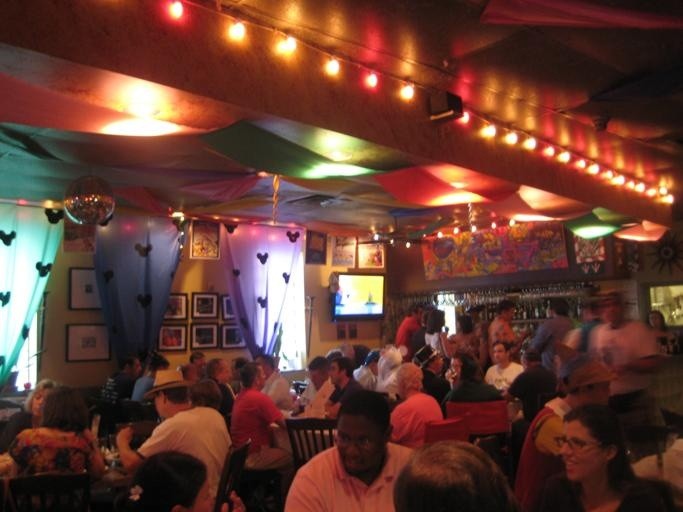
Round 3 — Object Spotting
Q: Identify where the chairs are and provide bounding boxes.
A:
[0,380,682,512]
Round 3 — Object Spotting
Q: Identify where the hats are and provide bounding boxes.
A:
[551,339,619,392]
[610,390,673,438]
[415,344,438,369]
[142,369,188,400]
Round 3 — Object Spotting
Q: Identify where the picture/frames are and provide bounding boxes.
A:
[159,291,188,321]
[219,291,237,321]
[155,323,188,354]
[186,215,223,262]
[66,264,106,313]
[191,290,220,321]
[222,323,248,353]
[192,325,218,353]
[63,321,108,364]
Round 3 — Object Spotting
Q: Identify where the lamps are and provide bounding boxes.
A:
[425,89,464,126]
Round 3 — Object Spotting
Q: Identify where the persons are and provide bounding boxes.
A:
[0,279,682,512]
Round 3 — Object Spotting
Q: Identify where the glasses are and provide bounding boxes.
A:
[333,428,370,448]
[554,436,600,450]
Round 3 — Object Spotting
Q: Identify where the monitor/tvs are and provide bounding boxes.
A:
[331,273,385,320]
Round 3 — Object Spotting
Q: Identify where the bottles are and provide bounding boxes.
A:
[401,282,574,308]
[488,299,552,320]
[575,297,582,319]
